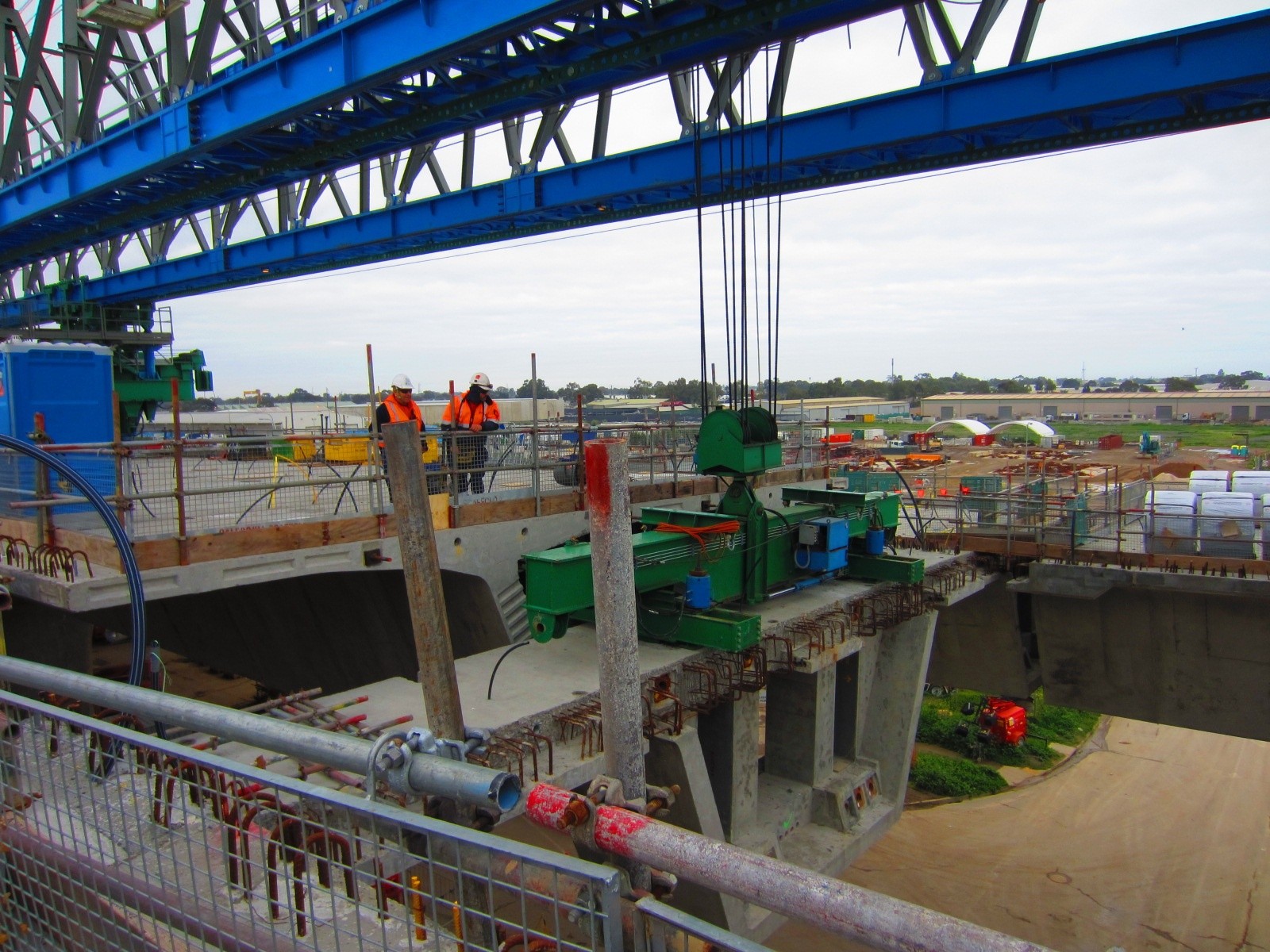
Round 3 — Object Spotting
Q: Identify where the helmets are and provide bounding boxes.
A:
[470,372,492,391]
[391,373,415,389]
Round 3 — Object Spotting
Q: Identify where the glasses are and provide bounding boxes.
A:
[478,387,488,393]
[397,389,412,393]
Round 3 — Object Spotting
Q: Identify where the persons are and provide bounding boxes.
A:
[368,373,428,502]
[441,372,500,496]
